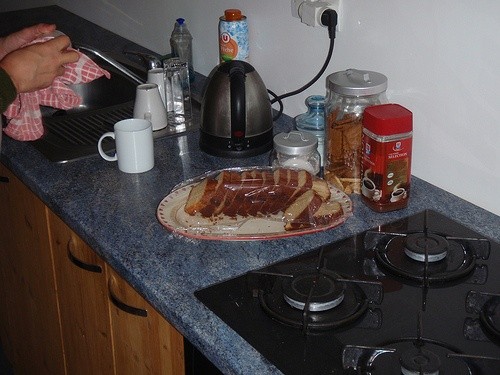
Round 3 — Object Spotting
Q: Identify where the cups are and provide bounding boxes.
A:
[162,58,193,132]
[146,68,173,112]
[133,83,168,132]
[98,118,155,174]
[34,30,72,49]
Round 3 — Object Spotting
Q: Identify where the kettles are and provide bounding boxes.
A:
[198,60,273,159]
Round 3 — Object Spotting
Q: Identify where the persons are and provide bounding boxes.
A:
[0,22,80,113]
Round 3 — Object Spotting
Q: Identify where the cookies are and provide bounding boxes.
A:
[326,108,363,193]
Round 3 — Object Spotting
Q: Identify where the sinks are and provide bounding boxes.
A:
[37,58,149,119]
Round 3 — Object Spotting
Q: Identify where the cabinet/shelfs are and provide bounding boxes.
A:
[0,163,68,374]
[44,202,187,375]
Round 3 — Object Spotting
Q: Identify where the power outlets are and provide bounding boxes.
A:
[290,0,341,30]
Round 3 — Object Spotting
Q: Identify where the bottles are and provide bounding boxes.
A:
[169,18,194,77]
[294,95,324,167]
[218,10,249,63]
[269,129,322,177]
[325,68,388,196]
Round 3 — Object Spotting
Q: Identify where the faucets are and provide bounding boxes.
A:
[72,41,163,85]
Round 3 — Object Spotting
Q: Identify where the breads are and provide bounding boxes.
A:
[185,167,343,231]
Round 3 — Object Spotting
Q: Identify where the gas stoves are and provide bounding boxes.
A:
[194,207,500,375]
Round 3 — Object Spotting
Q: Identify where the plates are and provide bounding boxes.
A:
[156,179,353,241]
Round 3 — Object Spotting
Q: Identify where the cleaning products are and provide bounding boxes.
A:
[169,18,195,81]
